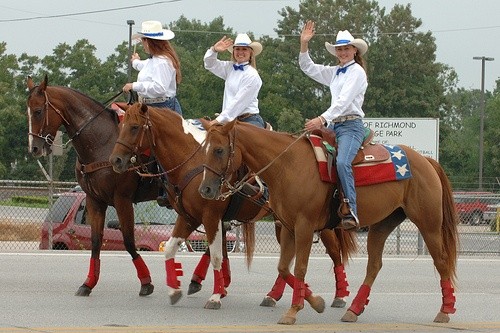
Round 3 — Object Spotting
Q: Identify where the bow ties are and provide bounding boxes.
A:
[233,63,249,71]
[335,62,356,75]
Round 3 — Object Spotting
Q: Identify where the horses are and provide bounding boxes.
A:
[109,97,349,311]
[197,115,463,324]
[25,74,217,296]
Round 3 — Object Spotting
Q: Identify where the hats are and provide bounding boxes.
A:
[137,21,175,40]
[227,34,262,56]
[325,29,368,57]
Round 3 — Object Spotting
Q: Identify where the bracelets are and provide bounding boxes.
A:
[318,116,324,125]
[213,46,217,52]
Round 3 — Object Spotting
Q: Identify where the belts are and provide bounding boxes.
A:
[138,97,169,103]
[238,113,253,119]
[334,115,359,122]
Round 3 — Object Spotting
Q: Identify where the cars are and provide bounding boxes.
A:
[39,191,237,253]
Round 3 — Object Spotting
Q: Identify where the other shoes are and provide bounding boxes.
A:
[343,218,356,229]
[157,196,172,209]
[243,183,267,204]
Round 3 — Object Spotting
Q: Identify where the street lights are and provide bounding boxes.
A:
[473,56,495,191]
[127,20,135,83]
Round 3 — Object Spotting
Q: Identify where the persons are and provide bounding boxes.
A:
[299,20,368,231]
[123,21,182,210]
[204,33,264,129]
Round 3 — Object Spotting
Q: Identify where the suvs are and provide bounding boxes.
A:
[452,191,500,225]
